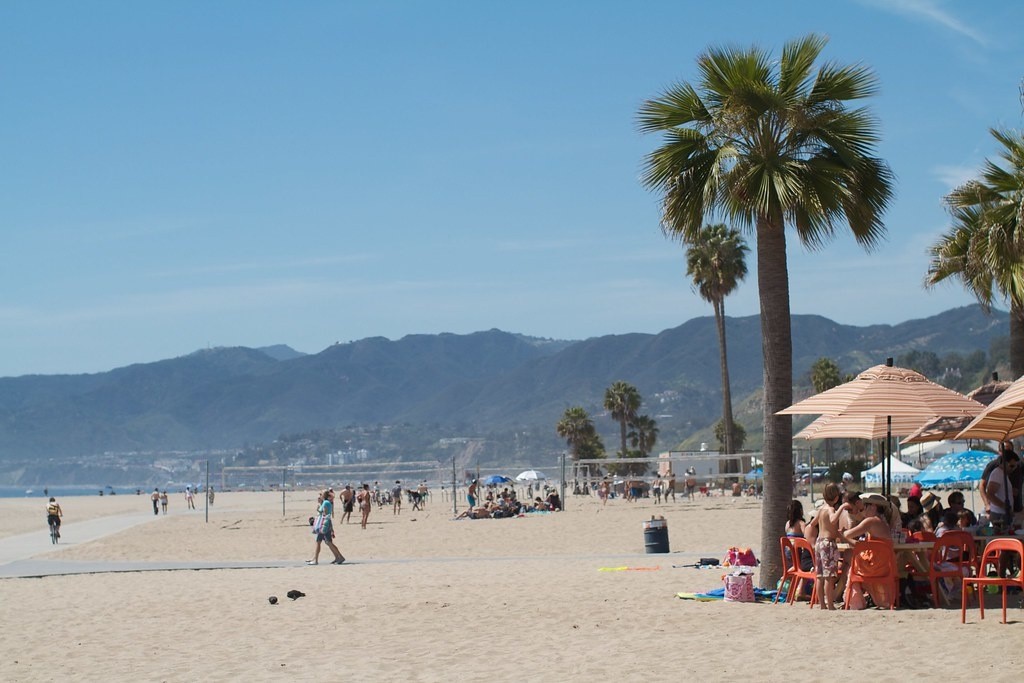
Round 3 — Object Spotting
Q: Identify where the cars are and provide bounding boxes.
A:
[793,467,832,486]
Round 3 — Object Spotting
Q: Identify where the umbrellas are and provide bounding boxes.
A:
[794,415,981,497]
[516,470,546,481]
[898,375,1024,445]
[484,475,513,484]
[773,354,989,506]
[912,447,1001,515]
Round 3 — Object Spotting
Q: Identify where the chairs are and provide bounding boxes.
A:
[774,528,1024,624]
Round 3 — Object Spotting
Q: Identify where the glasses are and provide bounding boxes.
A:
[953,500,964,505]
[1009,464,1018,468]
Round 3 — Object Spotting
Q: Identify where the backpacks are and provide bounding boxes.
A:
[48,504,59,516]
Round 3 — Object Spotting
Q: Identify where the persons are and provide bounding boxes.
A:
[308,489,345,566]
[208,487,216,504]
[783,473,1023,609]
[340,466,765,530]
[185,486,196,511]
[46,497,63,538]
[980,440,1024,593]
[160,491,169,515]
[150,488,162,514]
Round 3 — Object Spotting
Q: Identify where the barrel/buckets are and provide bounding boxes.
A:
[642,518,669,554]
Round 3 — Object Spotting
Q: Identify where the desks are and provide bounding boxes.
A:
[834,541,950,605]
[969,534,1023,578]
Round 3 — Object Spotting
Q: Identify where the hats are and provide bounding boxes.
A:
[918,492,941,514]
[472,479,477,484]
[861,494,890,514]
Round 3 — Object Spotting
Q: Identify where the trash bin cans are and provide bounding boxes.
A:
[642,520,670,553]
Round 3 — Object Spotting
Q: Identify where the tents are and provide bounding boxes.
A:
[859,455,922,487]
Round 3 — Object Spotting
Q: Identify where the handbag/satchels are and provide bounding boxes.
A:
[722,571,757,603]
[723,546,759,567]
[312,512,332,535]
